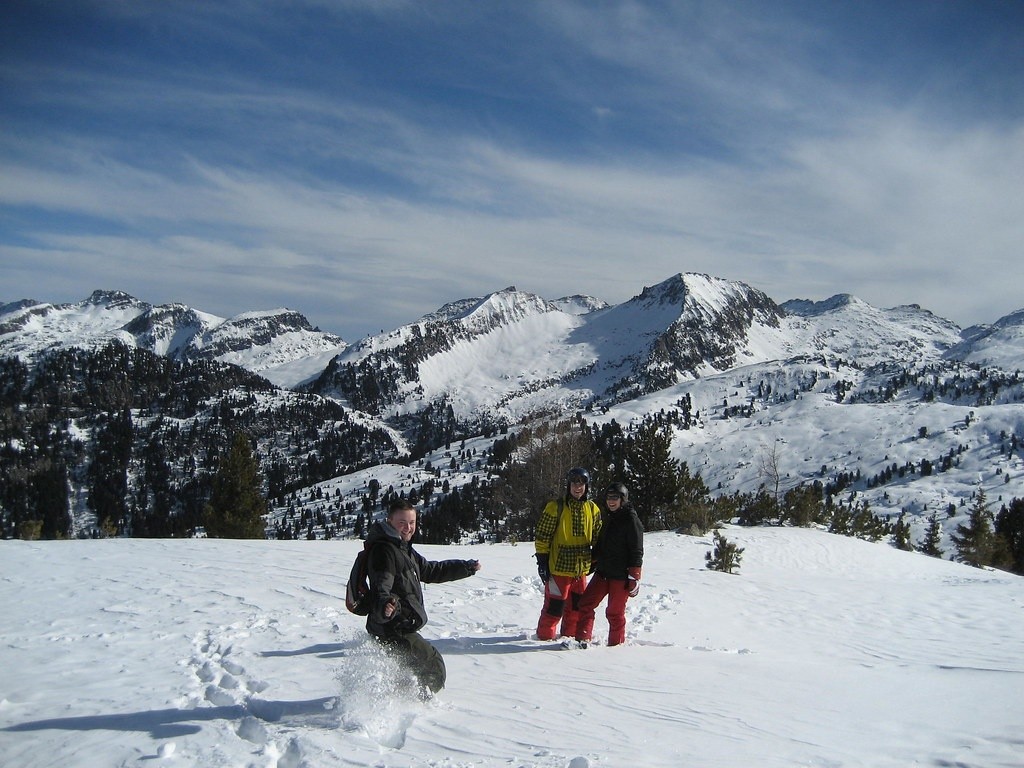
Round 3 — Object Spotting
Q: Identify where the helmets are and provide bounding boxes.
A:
[566,467,590,485]
[605,482,628,499]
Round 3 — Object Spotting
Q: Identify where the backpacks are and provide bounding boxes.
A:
[345,550,375,616]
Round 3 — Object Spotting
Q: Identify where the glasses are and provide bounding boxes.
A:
[606,495,621,501]
[569,474,589,483]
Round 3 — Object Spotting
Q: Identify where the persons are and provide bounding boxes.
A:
[365,501,481,705]
[535,468,602,641]
[561,482,644,649]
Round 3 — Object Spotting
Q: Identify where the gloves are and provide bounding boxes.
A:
[535,553,550,585]
[626,567,641,596]
